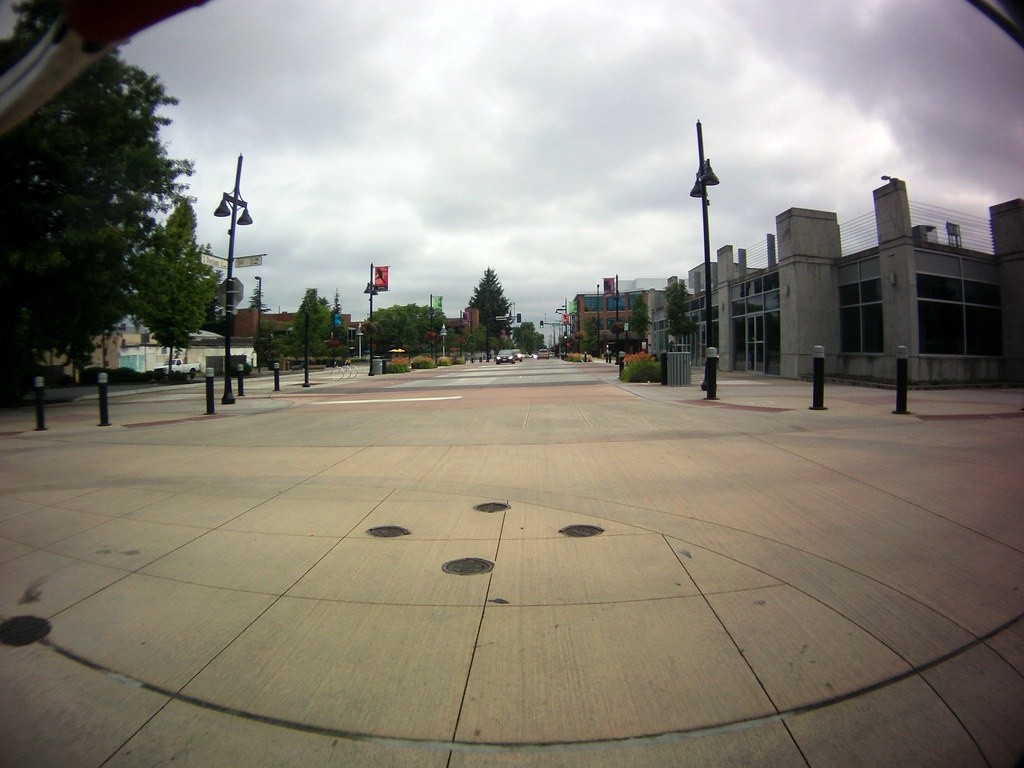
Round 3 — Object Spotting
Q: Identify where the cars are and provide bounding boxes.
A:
[510,348,523,362]
[549,351,555,358]
[495,349,515,365]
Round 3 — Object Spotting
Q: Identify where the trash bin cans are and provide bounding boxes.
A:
[372,356,383,375]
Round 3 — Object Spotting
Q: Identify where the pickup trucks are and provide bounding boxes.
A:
[154,359,200,378]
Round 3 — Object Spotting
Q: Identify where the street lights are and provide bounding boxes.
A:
[363,262,379,377]
[428,294,435,359]
[595,284,611,358]
[613,274,621,365]
[556,298,568,359]
[213,153,253,405]
[690,119,721,390]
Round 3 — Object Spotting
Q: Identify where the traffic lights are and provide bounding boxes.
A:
[540,321,543,328]
[563,332,568,339]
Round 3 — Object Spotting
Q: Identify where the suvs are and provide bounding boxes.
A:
[538,348,549,360]
